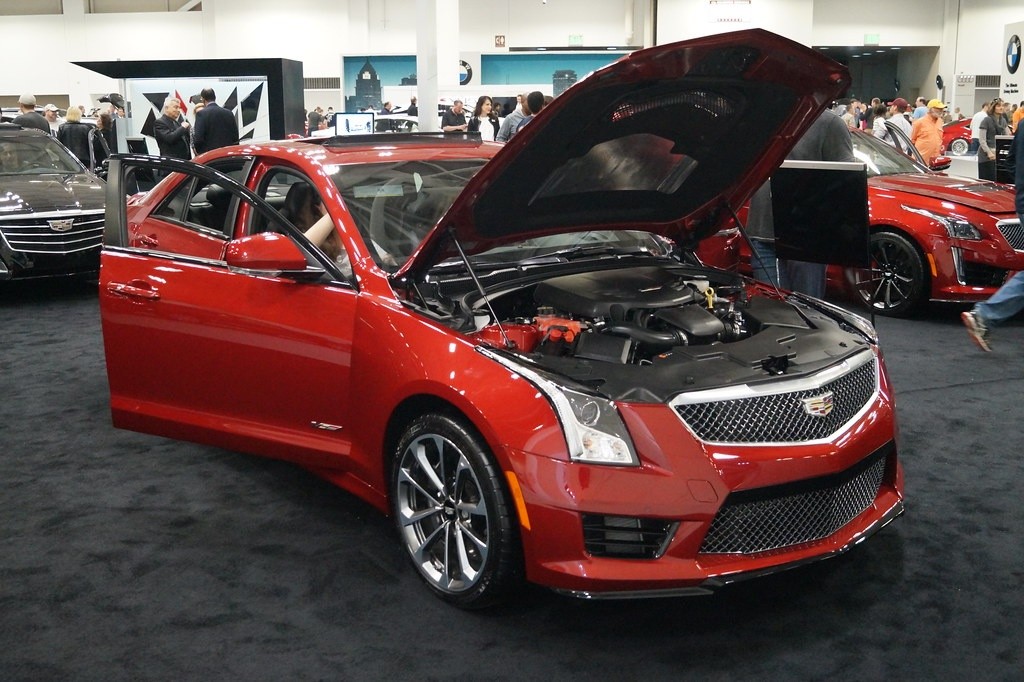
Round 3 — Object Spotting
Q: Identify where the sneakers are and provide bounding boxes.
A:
[961,307,994,352]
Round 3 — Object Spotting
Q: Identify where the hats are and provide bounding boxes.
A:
[45,104,60,112]
[887,98,908,112]
[17,94,37,105]
[927,99,948,108]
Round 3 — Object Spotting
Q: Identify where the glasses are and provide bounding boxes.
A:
[991,97,1001,105]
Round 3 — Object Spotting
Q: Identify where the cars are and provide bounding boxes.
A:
[99,26,907,620]
[938,116,1015,157]
[610,92,1024,319]
[0,106,112,301]
[311,96,506,138]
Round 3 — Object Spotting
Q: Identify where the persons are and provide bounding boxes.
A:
[305,107,335,137]
[1002,101,1024,134]
[468,96,500,141]
[905,97,961,123]
[407,96,418,132]
[154,99,192,177]
[361,105,373,112]
[291,185,394,281]
[744,112,853,300]
[875,98,913,156]
[978,98,1011,181]
[12,93,124,182]
[496,91,554,144]
[442,100,467,139]
[970,103,989,156]
[961,117,1024,351]
[911,98,949,168]
[0,143,31,172]
[375,102,393,132]
[829,98,891,135]
[189,88,243,195]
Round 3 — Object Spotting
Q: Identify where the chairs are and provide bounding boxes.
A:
[198,184,232,232]
[268,182,310,234]
